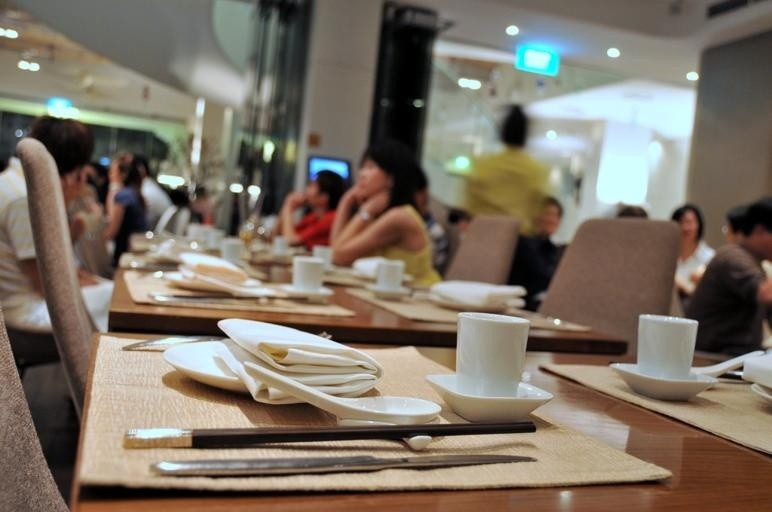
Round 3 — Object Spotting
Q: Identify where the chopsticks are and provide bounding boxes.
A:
[123,418,541,452]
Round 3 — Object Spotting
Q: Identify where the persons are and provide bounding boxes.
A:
[1,113,213,360]
[268,104,771,360]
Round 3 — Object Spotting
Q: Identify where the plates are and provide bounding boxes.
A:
[351,269,375,281]
[162,341,249,393]
[363,284,413,300]
[749,382,772,400]
[280,283,335,301]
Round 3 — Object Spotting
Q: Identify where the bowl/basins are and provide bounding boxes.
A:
[428,370,556,422]
[609,361,719,403]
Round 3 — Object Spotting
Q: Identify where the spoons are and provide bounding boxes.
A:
[243,361,442,425]
[692,349,762,379]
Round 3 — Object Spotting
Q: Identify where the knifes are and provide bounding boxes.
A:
[122,329,336,351]
[153,452,540,476]
[154,294,287,307]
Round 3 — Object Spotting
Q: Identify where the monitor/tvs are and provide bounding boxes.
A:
[303,155,349,181]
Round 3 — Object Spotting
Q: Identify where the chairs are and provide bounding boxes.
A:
[544,214,681,355]
[0,317,68,511]
[14,136,96,415]
[444,213,519,282]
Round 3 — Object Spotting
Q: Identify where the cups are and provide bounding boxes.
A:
[636,313,698,376]
[190,223,243,261]
[311,246,333,267]
[448,309,532,393]
[291,255,324,290]
[272,236,288,254]
[376,260,405,288]
